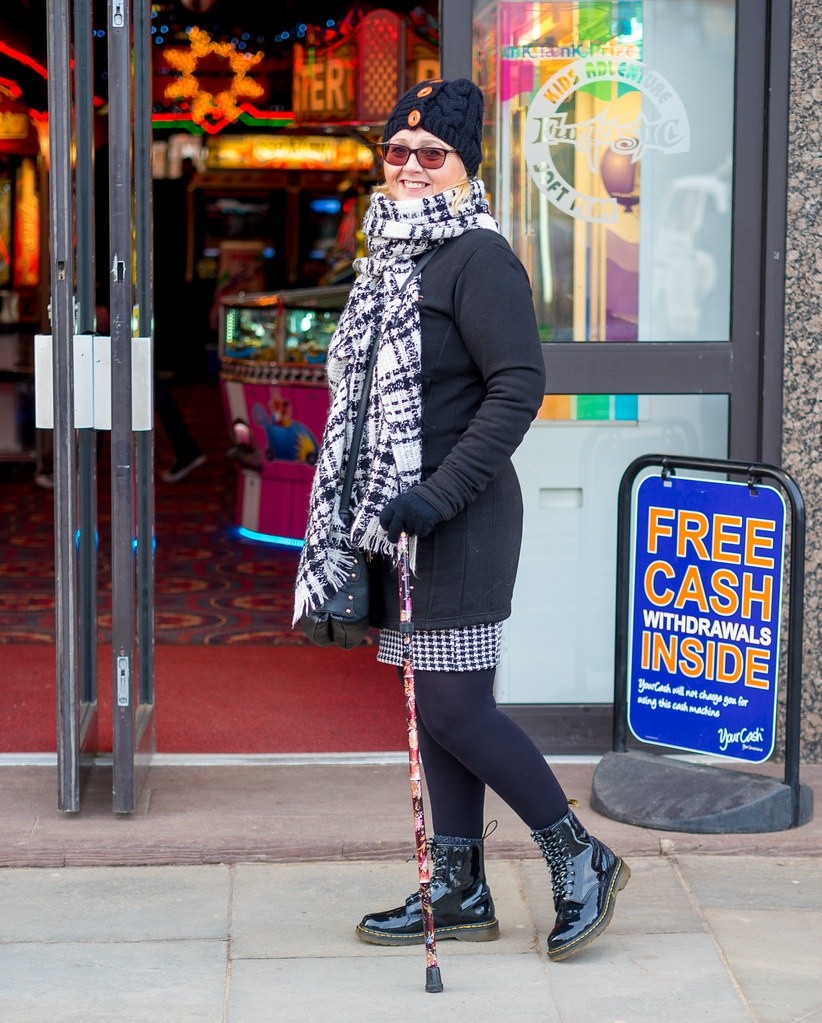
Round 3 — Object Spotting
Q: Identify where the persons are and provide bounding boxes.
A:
[35,142,207,489]
[210,263,265,326]
[292,78,632,961]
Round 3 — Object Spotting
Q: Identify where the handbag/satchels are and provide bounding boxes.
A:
[299,536,370,650]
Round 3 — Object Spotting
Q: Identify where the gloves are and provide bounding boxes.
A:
[380,491,443,544]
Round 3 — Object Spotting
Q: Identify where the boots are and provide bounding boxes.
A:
[356,819,500,946]
[530,798,631,961]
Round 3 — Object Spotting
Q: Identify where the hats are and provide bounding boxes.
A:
[381,78,484,178]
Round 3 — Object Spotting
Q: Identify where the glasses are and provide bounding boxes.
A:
[381,143,458,170]
